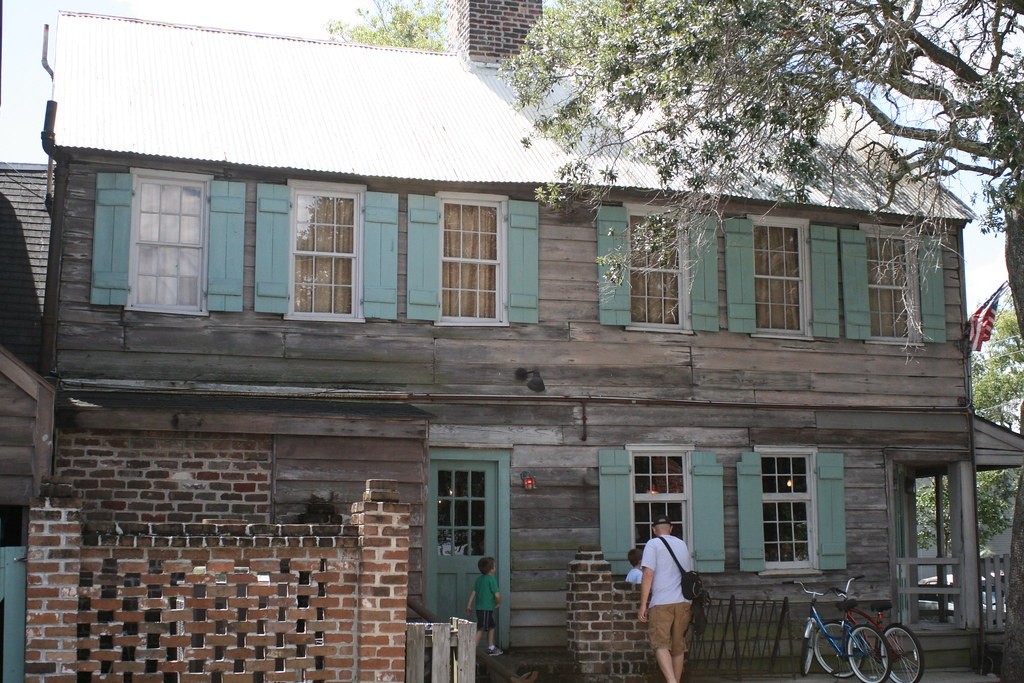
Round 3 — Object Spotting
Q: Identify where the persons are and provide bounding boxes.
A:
[466,556,504,666]
[638,514,694,683]
[625,548,643,584]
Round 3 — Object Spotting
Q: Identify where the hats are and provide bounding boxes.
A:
[654,515,672,527]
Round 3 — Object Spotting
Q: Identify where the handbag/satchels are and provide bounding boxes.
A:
[681,572,703,600]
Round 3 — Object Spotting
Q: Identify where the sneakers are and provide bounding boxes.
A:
[487,646,503,657]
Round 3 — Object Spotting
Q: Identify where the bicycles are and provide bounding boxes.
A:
[814,574,925,683]
[781,573,893,682]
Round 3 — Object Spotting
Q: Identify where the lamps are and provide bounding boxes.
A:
[520,471,536,490]
[907,477,916,495]
[515,367,545,393]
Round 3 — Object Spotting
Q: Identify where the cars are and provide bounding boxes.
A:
[918,572,1007,612]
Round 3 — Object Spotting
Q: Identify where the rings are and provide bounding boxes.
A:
[641,616,642,618]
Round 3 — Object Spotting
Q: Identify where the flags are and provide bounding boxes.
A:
[969,281,1007,351]
